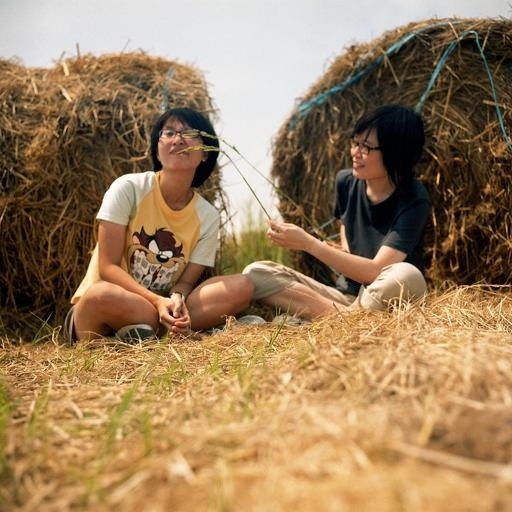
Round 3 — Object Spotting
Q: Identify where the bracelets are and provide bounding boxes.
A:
[169,289,185,298]
[170,287,186,299]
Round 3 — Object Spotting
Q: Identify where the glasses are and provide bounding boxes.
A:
[159,129,200,139]
[350,139,379,155]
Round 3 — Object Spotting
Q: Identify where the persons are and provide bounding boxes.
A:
[241,104,428,326]
[65,108,265,342]
